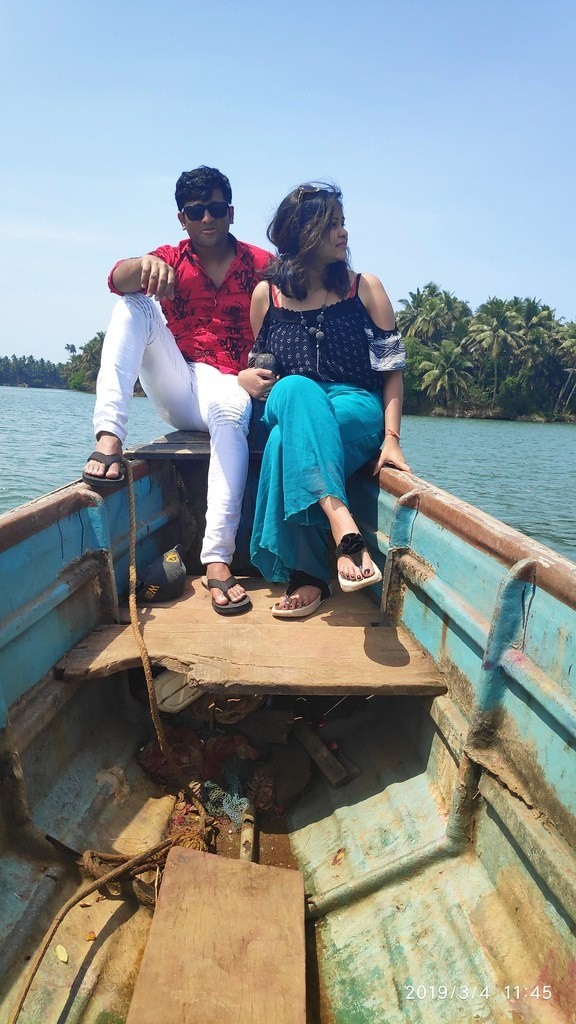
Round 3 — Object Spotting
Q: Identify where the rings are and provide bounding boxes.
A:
[264,392,269,398]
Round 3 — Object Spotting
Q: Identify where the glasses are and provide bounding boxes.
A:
[179,202,231,221]
[296,184,343,204]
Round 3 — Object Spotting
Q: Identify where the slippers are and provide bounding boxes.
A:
[272,571,337,620]
[83,452,132,487]
[200,573,253,616]
[334,531,382,592]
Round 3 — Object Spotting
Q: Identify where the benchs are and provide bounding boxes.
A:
[129,431,275,549]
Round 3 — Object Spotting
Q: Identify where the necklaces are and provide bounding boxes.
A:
[297,265,332,382]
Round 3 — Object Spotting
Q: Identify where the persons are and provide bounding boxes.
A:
[81,165,413,618]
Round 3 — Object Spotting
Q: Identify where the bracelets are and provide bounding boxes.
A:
[384,429,400,439]
